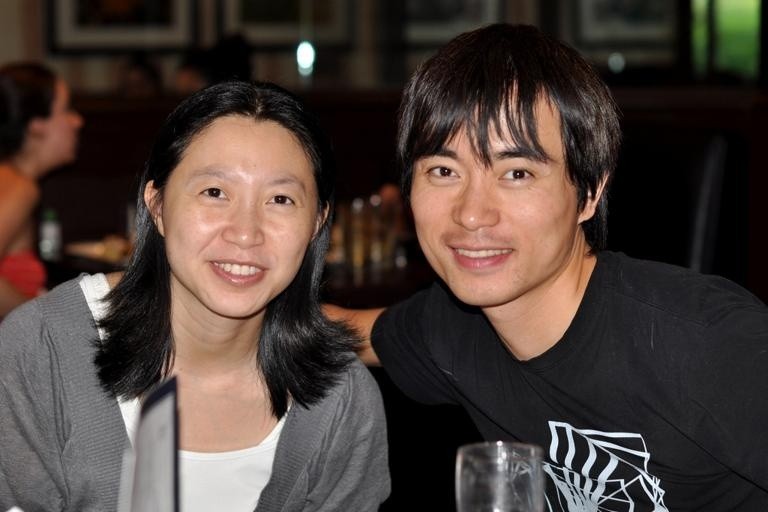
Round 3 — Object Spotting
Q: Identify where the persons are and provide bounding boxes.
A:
[318,21,767,511]
[0,79,393,511]
[0,59,85,320]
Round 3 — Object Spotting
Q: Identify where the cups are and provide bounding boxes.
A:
[453,441,544,512]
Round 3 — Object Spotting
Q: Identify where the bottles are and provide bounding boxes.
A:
[341,195,387,268]
[36,206,64,267]
